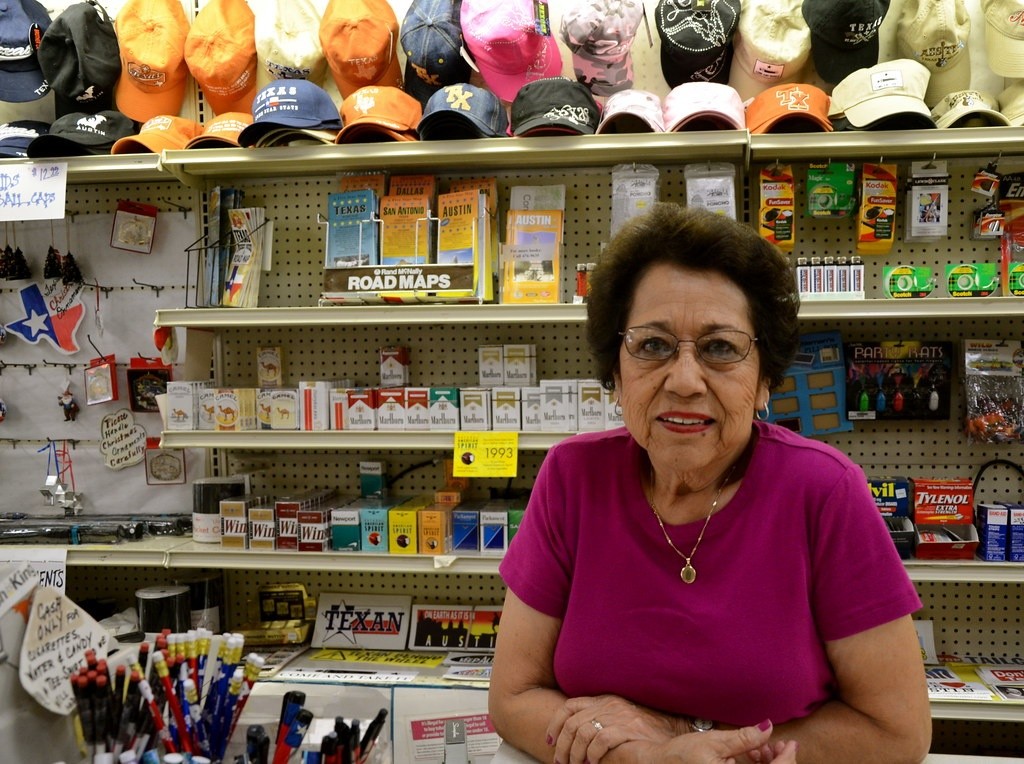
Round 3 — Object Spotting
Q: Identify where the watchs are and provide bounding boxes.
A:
[689,718,717,732]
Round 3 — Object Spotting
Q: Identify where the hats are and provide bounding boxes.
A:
[560,0,645,98]
[728,0,811,103]
[460,0,563,104]
[319,0,403,101]
[996,80,1024,126]
[930,90,1011,129]
[0,58,939,158]
[252,0,328,94]
[400,0,476,116]
[981,0,1024,79]
[37,0,122,113]
[801,0,890,86]
[184,0,256,116]
[655,0,741,89]
[896,0,972,107]
[115,0,190,123]
[0,0,51,103]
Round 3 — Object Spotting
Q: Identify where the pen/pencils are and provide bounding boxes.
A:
[71,627,388,764]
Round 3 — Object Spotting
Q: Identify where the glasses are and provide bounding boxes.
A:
[618,326,759,364]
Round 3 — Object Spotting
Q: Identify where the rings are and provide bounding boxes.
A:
[591,719,603,731]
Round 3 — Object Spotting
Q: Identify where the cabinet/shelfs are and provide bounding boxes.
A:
[161,130,747,575]
[749,127,1024,720]
[0,151,190,568]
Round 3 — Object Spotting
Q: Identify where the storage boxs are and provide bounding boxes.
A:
[169,345,625,435]
[868,476,910,519]
[886,518,914,560]
[1002,502,1024,561]
[219,460,528,556]
[907,477,974,525]
[976,504,1007,561]
[915,524,980,560]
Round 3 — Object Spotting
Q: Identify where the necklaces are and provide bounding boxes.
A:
[650,463,737,585]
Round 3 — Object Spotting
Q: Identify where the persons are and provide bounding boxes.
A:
[488,202,932,764]
[59,391,79,421]
[1005,687,1024,699]
